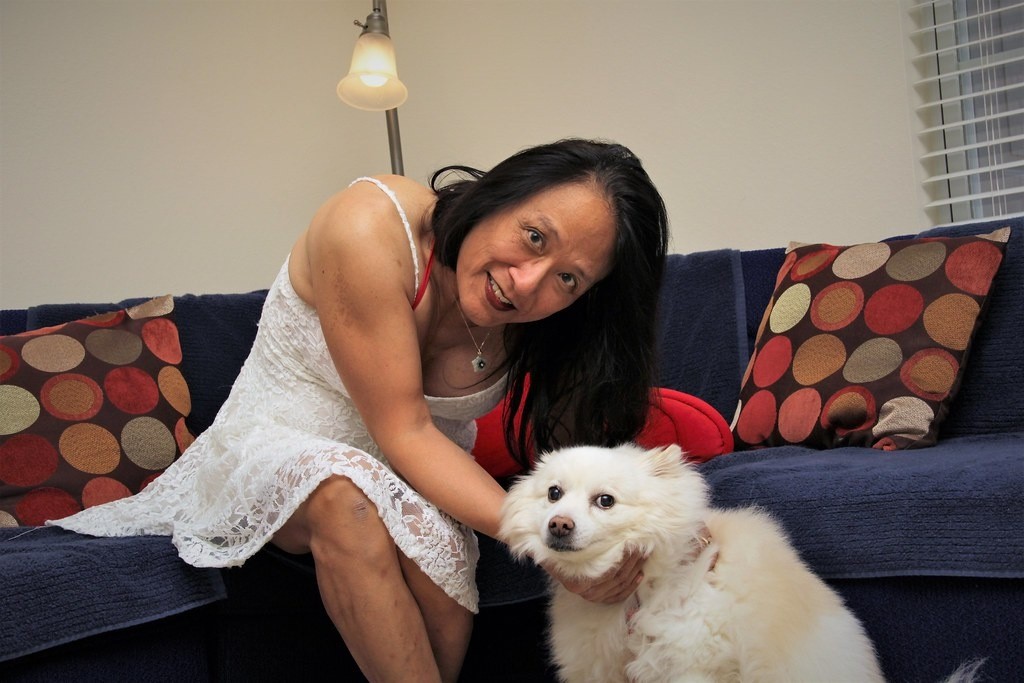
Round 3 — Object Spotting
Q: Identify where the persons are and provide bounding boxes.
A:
[42,139,670,681]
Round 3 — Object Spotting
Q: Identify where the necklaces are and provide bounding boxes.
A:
[444,272,494,372]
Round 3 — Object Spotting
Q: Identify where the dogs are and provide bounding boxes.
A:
[492,440,887,682]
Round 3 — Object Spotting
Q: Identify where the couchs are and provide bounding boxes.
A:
[1,217,1024,683]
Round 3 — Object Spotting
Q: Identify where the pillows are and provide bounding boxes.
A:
[729,225,1010,451]
[0,295,199,527]
[473,370,733,482]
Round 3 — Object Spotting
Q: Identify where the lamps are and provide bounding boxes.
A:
[336,2,411,177]
